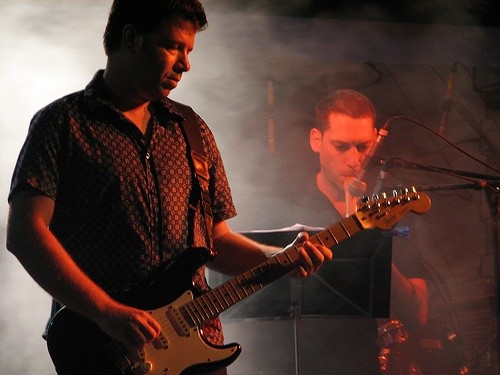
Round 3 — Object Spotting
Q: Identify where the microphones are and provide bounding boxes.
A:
[370,169,388,199]
[349,120,391,197]
[439,62,457,136]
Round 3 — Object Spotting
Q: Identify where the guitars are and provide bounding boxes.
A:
[46,186,432,375]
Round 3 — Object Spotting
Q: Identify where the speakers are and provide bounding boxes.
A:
[222,316,382,375]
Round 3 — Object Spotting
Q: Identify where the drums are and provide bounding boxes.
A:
[376,317,478,375]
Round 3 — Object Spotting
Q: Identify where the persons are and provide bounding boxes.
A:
[6,0,332,375]
[260,90,428,327]
